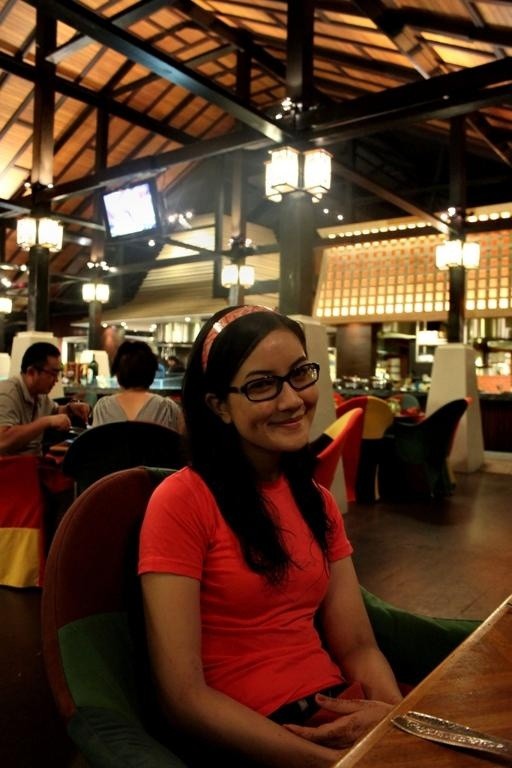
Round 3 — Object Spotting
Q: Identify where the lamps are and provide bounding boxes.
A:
[217,261,259,293]
[79,278,112,304]
[11,213,67,256]
[258,143,339,206]
[433,237,483,273]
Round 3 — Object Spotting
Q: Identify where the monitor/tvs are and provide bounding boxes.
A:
[99,177,167,247]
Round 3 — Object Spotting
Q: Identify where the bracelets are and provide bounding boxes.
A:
[66,402,74,417]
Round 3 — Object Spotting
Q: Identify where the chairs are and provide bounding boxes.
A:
[332,395,394,506]
[54,419,183,498]
[381,397,470,506]
[41,462,483,765]
[311,406,366,526]
[0,442,68,594]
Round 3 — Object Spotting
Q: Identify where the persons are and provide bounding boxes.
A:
[0,341,91,456]
[90,340,187,435]
[137,303,405,767]
[167,355,186,373]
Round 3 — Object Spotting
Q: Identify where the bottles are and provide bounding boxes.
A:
[80,367,87,389]
[87,368,94,383]
[89,353,98,375]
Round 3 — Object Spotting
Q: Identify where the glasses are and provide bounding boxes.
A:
[228,361,321,403]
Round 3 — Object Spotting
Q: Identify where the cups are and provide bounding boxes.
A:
[96,376,118,388]
